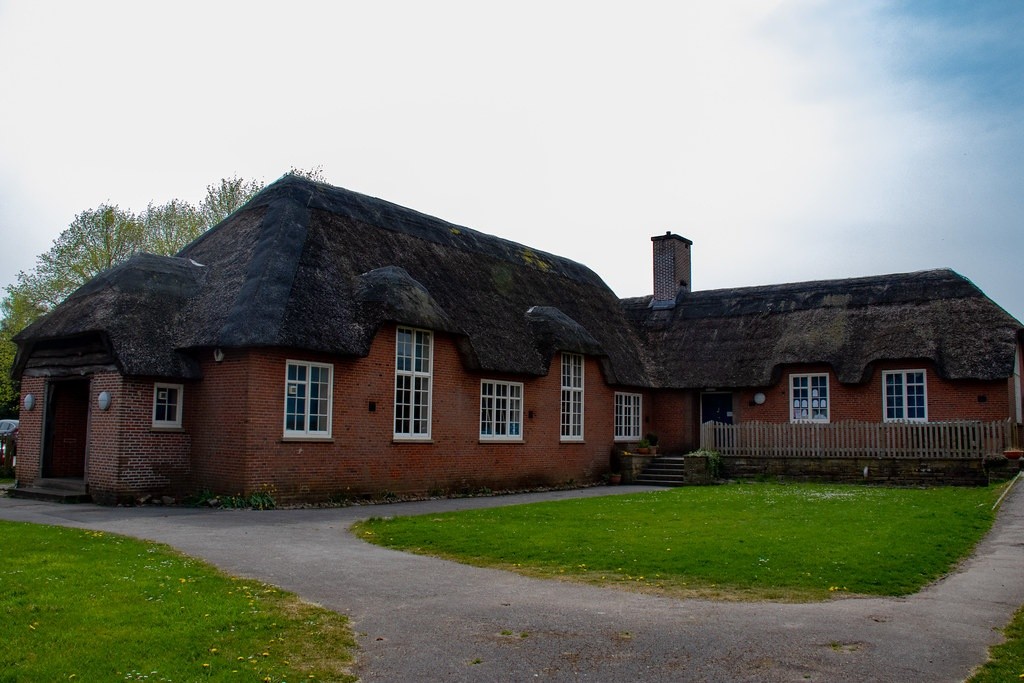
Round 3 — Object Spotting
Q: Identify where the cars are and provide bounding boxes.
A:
[0,420,19,440]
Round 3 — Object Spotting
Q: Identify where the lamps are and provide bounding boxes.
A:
[754,393,765,404]
[23,394,35,411]
[98,392,111,409]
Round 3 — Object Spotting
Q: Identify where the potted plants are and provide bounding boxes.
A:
[1004,448,1023,459]
[609,443,624,483]
[639,437,650,452]
[645,434,660,453]
